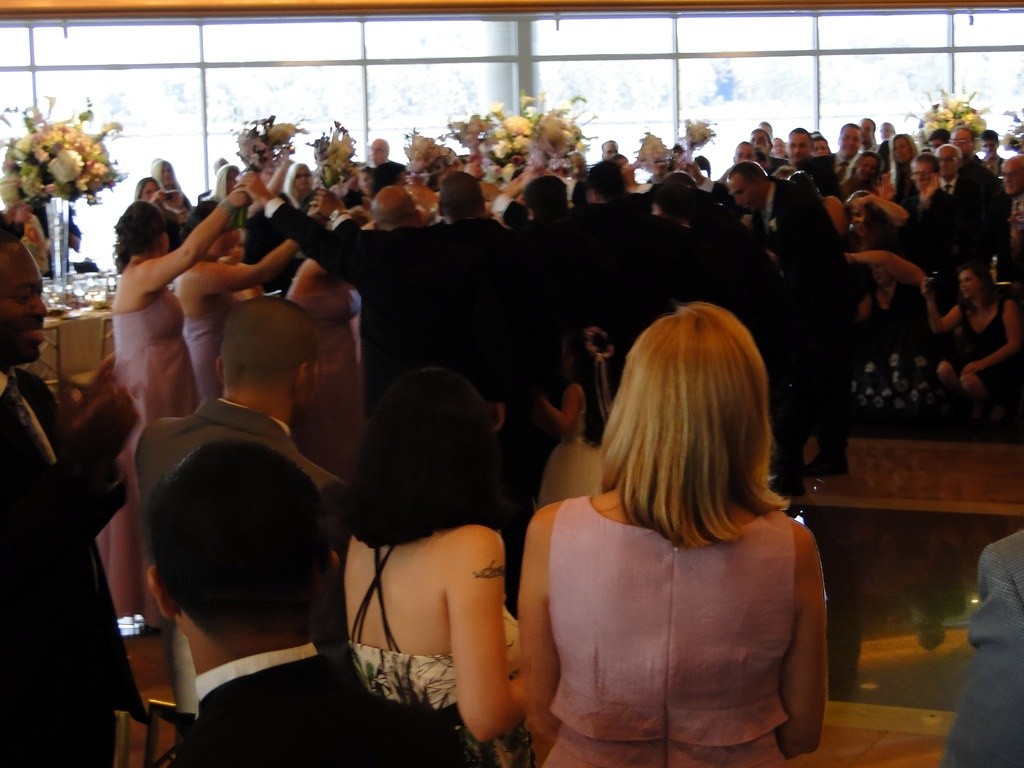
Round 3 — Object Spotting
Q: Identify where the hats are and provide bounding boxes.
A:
[371,367,506,448]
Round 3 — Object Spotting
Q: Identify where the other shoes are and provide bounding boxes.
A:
[805,452,849,475]
[771,478,806,496]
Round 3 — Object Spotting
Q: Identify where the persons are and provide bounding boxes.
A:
[336,364,536,768]
[136,116,1024,499]
[94,189,253,638]
[515,300,830,768]
[138,435,464,768]
[936,526,1024,768]
[1,215,149,768]
[135,295,345,705]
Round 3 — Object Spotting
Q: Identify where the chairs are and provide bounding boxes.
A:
[141,699,176,768]
[66,318,113,415]
[21,325,72,429]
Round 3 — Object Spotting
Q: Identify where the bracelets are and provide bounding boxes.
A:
[216,199,239,220]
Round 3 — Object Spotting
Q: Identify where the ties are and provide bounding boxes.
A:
[1,375,58,469]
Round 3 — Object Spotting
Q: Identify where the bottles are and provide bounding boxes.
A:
[233,202,250,249]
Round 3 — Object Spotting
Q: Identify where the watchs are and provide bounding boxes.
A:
[329,208,352,226]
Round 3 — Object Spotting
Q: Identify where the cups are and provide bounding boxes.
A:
[40,271,121,316]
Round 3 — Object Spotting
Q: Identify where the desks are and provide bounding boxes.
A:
[18,309,115,424]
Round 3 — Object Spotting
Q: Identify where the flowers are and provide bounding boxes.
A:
[632,126,678,174]
[678,119,718,164]
[904,85,994,155]
[402,126,458,185]
[0,95,130,278]
[436,85,600,191]
[996,108,1024,154]
[305,120,359,198]
[227,114,313,226]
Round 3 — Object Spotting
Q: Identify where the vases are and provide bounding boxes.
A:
[44,197,69,292]
